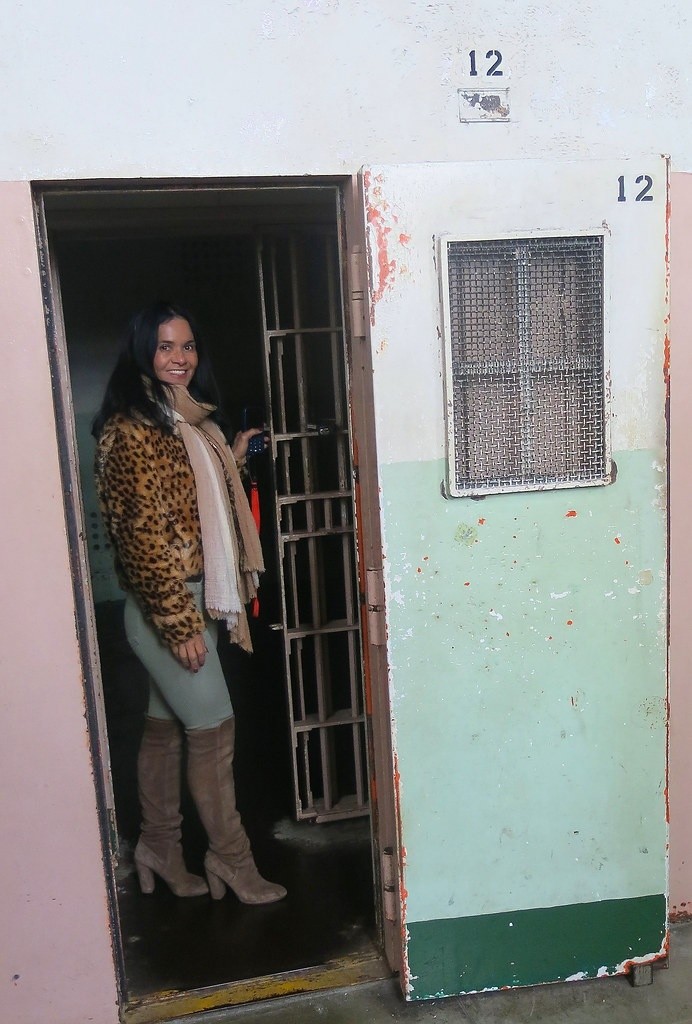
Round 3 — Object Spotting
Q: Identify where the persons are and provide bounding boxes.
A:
[88,298,291,906]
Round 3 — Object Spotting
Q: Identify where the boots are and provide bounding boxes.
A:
[186,717,288,904]
[132,702,212,897]
